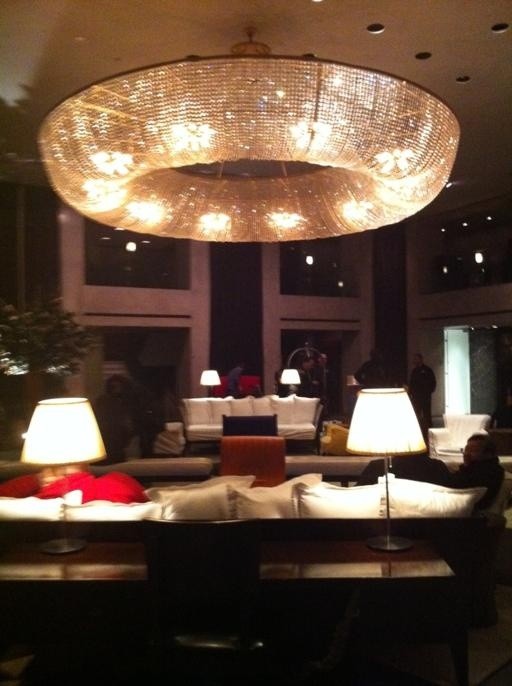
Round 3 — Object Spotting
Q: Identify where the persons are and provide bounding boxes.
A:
[313,354,329,398]
[407,353,436,427]
[354,349,388,388]
[489,388,512,430]
[227,361,245,396]
[446,434,505,497]
[296,358,314,398]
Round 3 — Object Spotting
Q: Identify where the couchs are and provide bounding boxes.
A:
[183,397,322,443]
[429,410,491,463]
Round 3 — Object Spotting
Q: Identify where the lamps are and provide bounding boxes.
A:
[37,55,461,242]
[19,396,106,555]
[201,370,220,400]
[280,368,302,396]
[345,387,426,553]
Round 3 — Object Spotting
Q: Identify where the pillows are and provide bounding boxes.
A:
[294,483,386,520]
[229,473,324,520]
[147,476,254,520]
[65,501,163,520]
[383,484,485,519]
[3,492,81,520]
